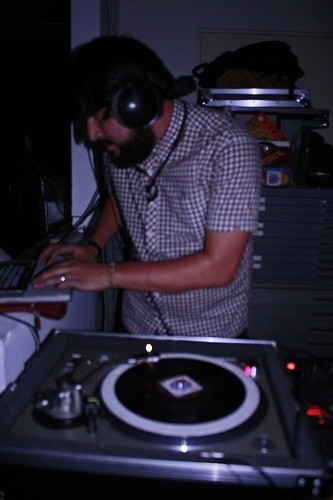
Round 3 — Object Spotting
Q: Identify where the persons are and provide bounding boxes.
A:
[29,35,262,339]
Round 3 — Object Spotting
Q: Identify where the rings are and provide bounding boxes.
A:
[61,274,66,284]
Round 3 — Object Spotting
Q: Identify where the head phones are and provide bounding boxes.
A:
[112,73,197,131]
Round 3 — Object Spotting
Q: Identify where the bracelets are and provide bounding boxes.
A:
[108,261,116,288]
[87,239,103,259]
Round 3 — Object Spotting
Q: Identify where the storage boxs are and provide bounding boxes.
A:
[196,87,311,108]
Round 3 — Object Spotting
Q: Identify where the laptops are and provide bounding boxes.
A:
[0,256,72,307]
